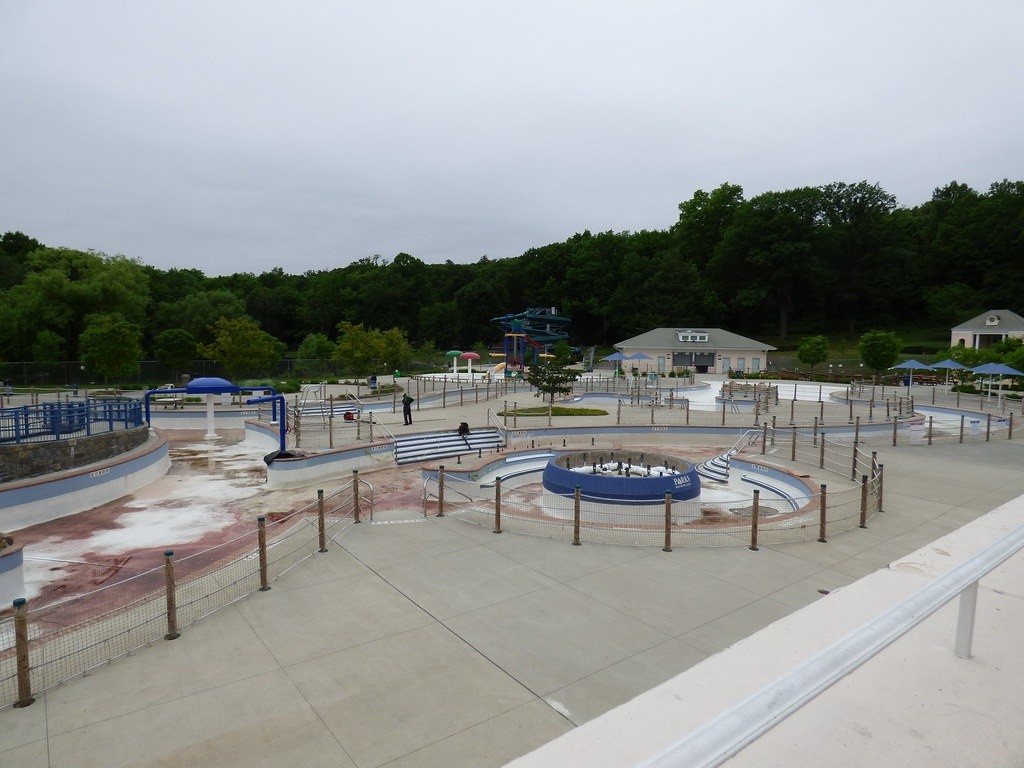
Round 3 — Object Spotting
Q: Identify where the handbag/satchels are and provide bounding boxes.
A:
[408,396,414,405]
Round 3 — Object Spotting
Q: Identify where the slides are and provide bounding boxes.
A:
[481,362,506,379]
[488,308,582,358]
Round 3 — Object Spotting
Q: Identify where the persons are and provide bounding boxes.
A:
[459,422,469,435]
[403,393,414,426]
[448,361,452,372]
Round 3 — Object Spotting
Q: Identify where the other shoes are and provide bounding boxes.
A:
[403,424,408,425]
[409,423,412,424]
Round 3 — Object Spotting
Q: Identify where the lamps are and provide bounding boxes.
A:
[666,353,670,359]
[717,355,722,360]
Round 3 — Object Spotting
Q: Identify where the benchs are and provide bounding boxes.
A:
[912,375,958,386]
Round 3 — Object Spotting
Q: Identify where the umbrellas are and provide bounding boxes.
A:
[891,359,1024,408]
[600,352,653,378]
